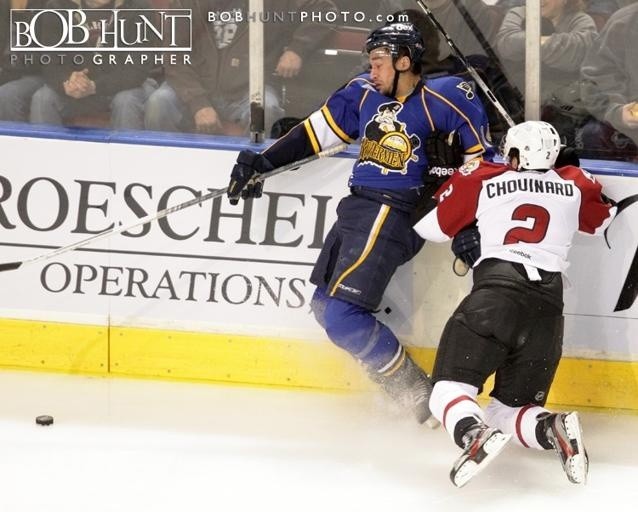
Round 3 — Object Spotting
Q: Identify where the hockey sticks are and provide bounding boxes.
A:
[0,137,348,272]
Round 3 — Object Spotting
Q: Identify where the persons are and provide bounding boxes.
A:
[225,20,496,428]
[424,115,621,485]
[2,2,638,164]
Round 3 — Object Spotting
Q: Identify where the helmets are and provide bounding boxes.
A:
[364,21,425,67]
[503,120,561,171]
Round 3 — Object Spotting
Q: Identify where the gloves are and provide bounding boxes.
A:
[227,148,275,205]
[451,226,481,269]
[425,128,462,185]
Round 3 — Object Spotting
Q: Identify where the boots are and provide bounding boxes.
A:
[535,411,589,484]
[450,413,506,487]
[367,340,434,424]
